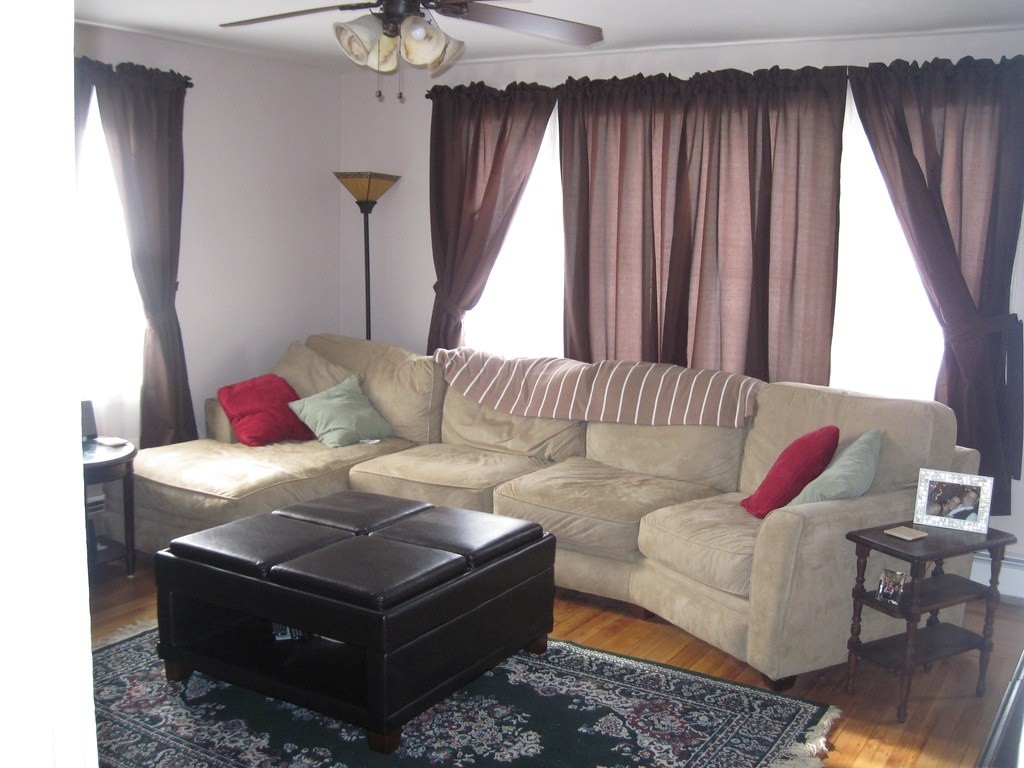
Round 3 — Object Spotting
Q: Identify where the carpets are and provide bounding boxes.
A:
[92,613,843,768]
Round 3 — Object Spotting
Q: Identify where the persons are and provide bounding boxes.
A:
[946,489,979,521]
[927,495,961,516]
[884,581,894,599]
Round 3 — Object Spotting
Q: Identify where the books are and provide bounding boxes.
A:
[93,437,127,446]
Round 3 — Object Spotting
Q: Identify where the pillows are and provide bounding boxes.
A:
[787,428,882,503]
[740,425,841,519]
[216,372,315,446]
[288,374,395,449]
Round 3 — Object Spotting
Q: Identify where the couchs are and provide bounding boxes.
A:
[98,334,979,692]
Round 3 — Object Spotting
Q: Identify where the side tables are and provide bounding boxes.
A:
[83,436,138,579]
[844,520,1018,723]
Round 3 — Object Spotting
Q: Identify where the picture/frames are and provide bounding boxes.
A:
[912,467,996,534]
[875,568,908,605]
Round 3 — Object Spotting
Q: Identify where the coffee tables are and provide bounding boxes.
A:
[155,489,558,750]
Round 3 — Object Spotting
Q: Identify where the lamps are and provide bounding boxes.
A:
[333,172,402,340]
[333,13,466,77]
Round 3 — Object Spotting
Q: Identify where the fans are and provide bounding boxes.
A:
[219,0,605,48]
